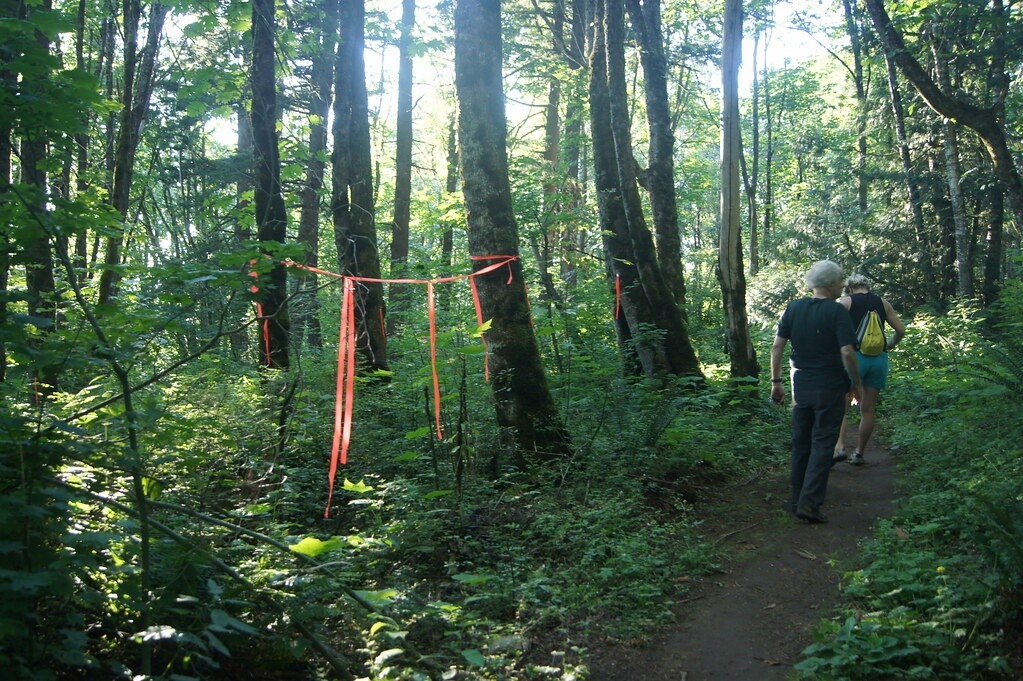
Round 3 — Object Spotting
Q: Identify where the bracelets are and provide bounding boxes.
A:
[771,378,782,383]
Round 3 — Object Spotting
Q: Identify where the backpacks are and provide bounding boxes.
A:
[856,296,888,355]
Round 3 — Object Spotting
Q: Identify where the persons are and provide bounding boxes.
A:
[831,273,907,465]
[771,259,864,524]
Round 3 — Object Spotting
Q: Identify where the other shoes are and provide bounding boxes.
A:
[831,446,849,466]
[847,452,865,465]
[795,503,830,523]
